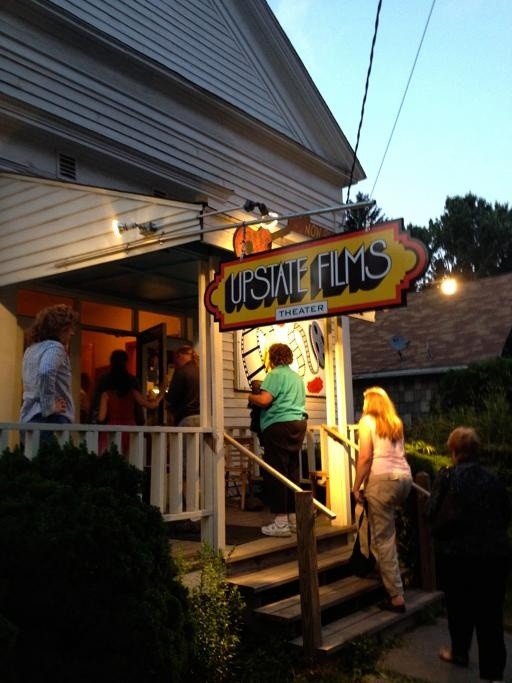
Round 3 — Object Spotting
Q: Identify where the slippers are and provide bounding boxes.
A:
[377,600,406,614]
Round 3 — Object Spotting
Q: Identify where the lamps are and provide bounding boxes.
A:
[114,221,157,235]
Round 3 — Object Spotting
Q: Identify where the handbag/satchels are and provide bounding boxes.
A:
[431,493,465,538]
[350,549,376,578]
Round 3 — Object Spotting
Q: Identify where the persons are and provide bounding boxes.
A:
[17,303,81,449]
[80,369,90,424]
[352,385,414,614]
[247,343,309,537]
[88,350,136,425]
[432,424,512,683]
[169,336,200,537]
[96,368,168,463]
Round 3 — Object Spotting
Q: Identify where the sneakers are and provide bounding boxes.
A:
[289,520,297,534]
[262,523,291,536]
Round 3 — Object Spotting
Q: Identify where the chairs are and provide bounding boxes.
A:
[307,425,331,520]
[224,436,264,510]
